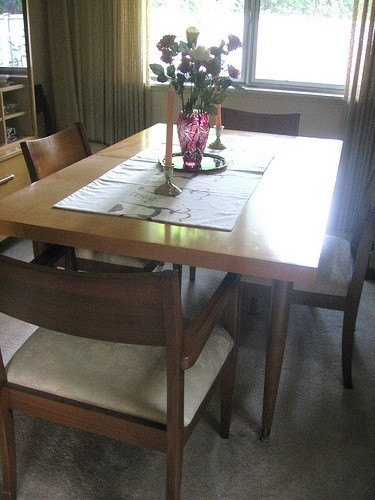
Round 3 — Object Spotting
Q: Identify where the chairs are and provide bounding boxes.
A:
[0,254,241,500]
[242,175,375,392]
[20,121,165,271]
[220,106,301,137]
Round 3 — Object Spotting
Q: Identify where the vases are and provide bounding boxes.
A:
[175,109,210,170]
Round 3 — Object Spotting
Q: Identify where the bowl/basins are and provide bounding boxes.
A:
[0,75,9,83]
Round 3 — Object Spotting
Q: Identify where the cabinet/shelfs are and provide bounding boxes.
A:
[0,0,40,243]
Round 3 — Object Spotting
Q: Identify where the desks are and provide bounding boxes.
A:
[0,121,344,444]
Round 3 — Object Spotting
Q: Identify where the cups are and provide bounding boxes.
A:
[6,127,16,138]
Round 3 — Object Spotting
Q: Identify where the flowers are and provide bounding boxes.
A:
[148,25,243,164]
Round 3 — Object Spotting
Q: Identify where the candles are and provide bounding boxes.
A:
[166,87,175,164]
[214,87,221,127]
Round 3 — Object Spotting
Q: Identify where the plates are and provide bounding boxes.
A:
[7,136,17,141]
